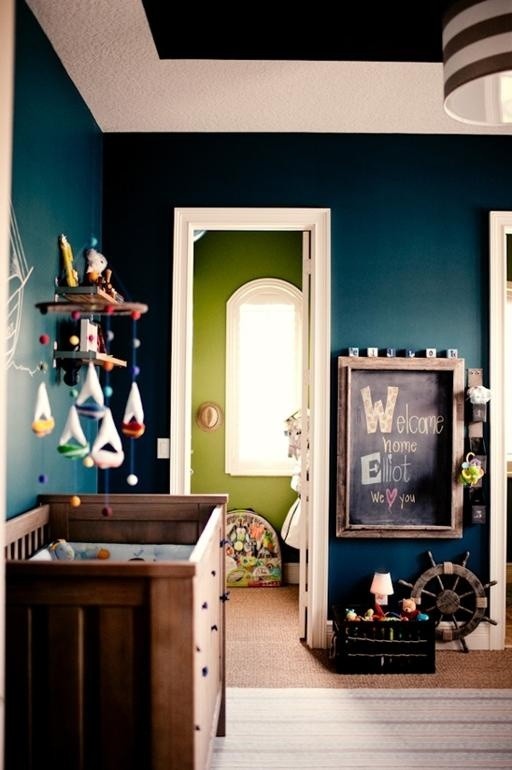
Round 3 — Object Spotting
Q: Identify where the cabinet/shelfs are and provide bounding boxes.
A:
[36,231,130,377]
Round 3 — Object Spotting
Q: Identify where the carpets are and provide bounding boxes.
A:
[190,687,512,770]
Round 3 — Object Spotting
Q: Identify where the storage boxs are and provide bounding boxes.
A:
[327,608,437,675]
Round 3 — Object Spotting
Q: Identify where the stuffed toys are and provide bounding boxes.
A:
[456,452,485,487]
[340,595,429,624]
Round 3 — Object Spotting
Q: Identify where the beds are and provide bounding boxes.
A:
[5,485,234,770]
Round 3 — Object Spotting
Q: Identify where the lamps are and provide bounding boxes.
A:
[441,0,512,142]
